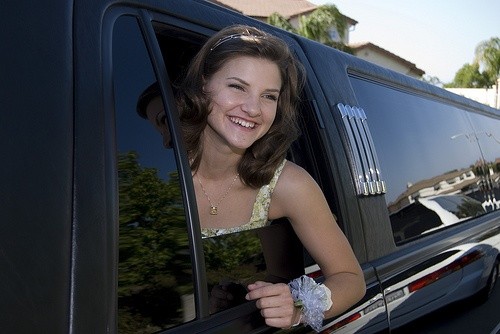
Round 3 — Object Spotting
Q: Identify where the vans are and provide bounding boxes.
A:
[0,2,500,334]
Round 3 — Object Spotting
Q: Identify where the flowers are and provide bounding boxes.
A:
[290,275,333,333]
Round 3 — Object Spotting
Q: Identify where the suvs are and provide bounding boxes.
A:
[390,193,487,250]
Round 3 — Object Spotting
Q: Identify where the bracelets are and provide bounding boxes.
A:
[285,274,335,333]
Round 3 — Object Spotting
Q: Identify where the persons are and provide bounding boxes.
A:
[134,80,174,155]
[177,22,367,327]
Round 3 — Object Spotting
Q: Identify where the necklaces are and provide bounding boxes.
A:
[184,150,241,217]
